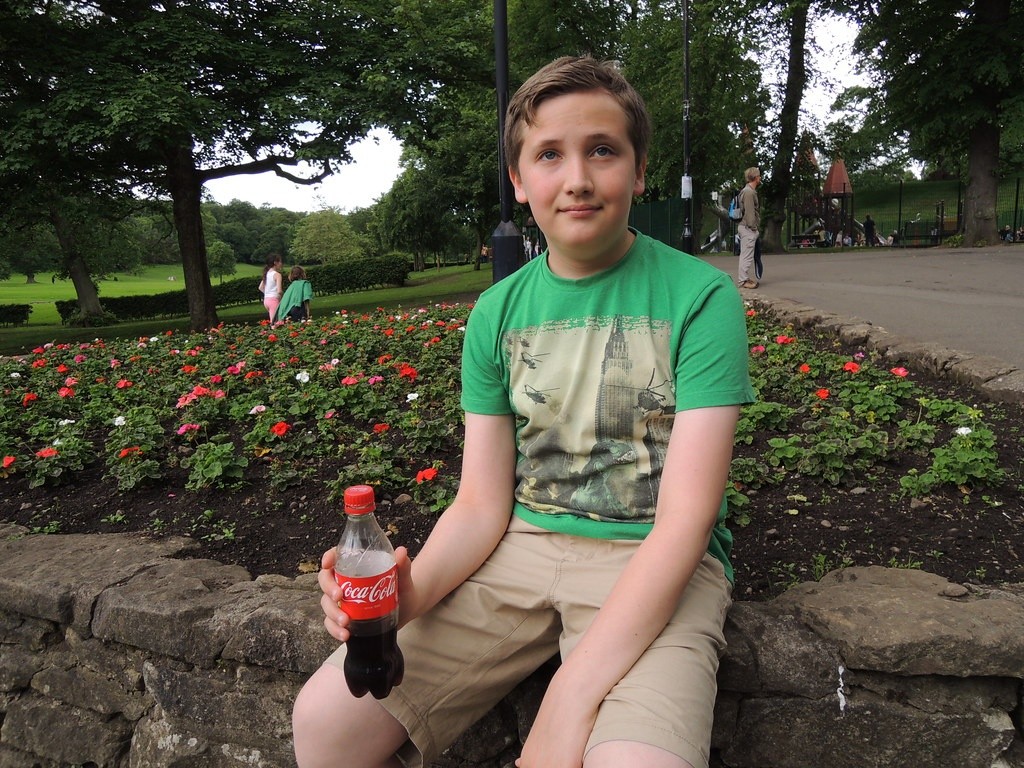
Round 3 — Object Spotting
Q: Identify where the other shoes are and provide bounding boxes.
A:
[757,274,761,279]
[739,281,759,289]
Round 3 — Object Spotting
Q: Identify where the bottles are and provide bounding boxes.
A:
[333,484,404,699]
[733,208,735,210]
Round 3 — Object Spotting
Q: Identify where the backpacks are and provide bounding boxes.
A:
[728,187,752,222]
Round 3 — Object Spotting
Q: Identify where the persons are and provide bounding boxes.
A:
[912,213,921,245]
[738,167,761,288]
[482,244,488,263]
[931,212,947,244]
[524,237,543,260]
[272,266,312,323]
[862,215,876,247]
[258,253,284,323]
[1000,225,1024,241]
[801,226,897,248]
[292,57,756,768]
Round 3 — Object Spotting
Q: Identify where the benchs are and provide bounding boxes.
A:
[900,221,944,249]
[793,234,820,249]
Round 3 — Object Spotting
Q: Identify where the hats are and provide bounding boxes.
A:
[1005,225,1010,230]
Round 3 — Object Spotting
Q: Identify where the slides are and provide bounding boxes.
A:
[786,221,824,247]
[825,200,887,246]
[699,182,733,251]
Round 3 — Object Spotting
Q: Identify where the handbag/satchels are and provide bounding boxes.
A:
[284,305,307,323]
[872,236,879,244]
